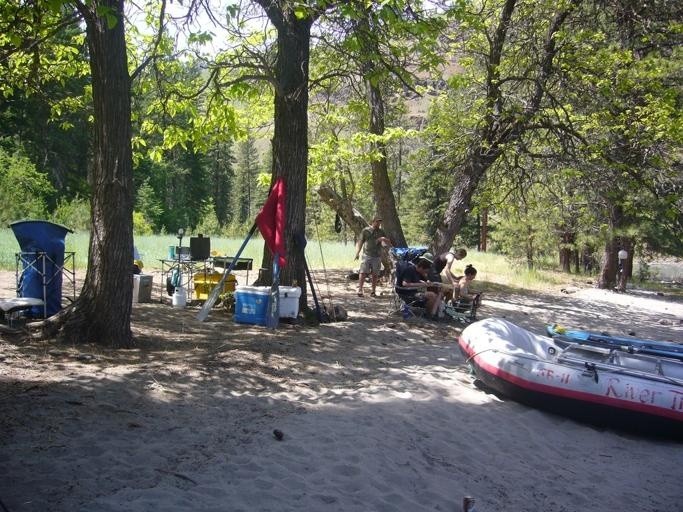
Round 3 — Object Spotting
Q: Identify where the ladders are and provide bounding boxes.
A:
[557,342,683,386]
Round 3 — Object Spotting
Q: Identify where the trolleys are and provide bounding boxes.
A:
[208,256,253,312]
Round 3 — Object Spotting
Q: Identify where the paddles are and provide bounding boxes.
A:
[267,254,279,327]
[295,231,327,323]
[196,224,257,321]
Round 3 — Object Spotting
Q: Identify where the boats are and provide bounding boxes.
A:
[458,316,682,438]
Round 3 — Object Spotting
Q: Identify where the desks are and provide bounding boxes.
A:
[155,258,217,304]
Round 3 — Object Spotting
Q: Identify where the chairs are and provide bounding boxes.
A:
[389,286,428,322]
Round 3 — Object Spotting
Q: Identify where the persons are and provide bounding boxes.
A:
[582,250,593,277]
[354,214,389,297]
[395,248,478,320]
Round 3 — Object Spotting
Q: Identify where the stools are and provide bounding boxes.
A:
[443,290,484,322]
[131,273,154,303]
